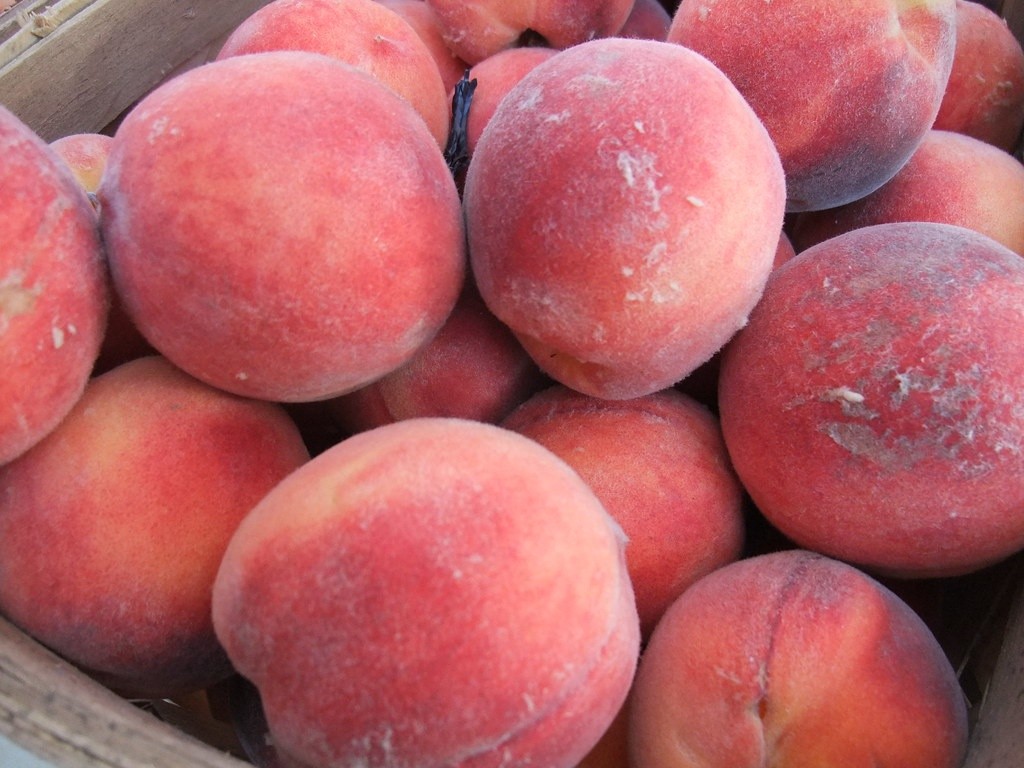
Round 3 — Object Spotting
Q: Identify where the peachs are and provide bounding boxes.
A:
[0,0,1024,768]
